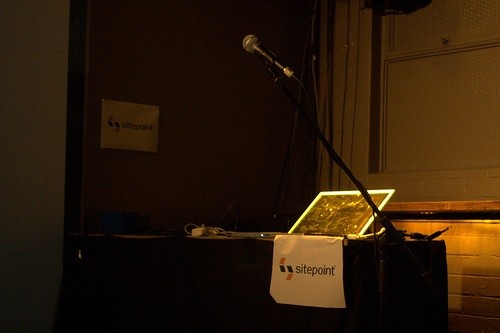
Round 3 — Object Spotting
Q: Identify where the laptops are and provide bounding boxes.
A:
[288,189,395,240]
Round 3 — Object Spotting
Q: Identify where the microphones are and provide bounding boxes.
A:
[243,35,300,82]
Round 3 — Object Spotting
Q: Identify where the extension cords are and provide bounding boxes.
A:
[192,226,218,236]
